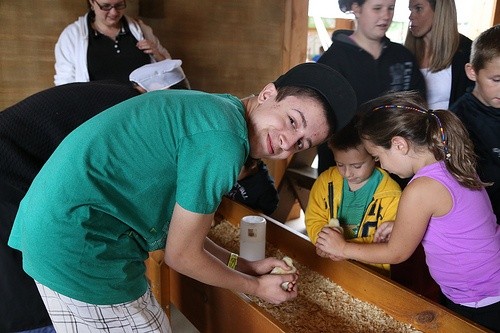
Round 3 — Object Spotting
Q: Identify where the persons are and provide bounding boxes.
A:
[0,59,190,333]
[316,93,500,333]
[404,0,500,224]
[305,124,402,280]
[227,155,279,217]
[316,0,428,295]
[53,0,172,86]
[7,62,358,333]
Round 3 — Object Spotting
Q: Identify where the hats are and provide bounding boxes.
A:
[129,59,186,92]
[274,62,357,131]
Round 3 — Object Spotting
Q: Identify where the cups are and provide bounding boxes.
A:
[239,216,266,262]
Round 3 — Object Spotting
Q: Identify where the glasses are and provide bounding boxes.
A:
[94,0,126,11]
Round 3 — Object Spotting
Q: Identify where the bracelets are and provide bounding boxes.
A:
[228,253,238,270]
